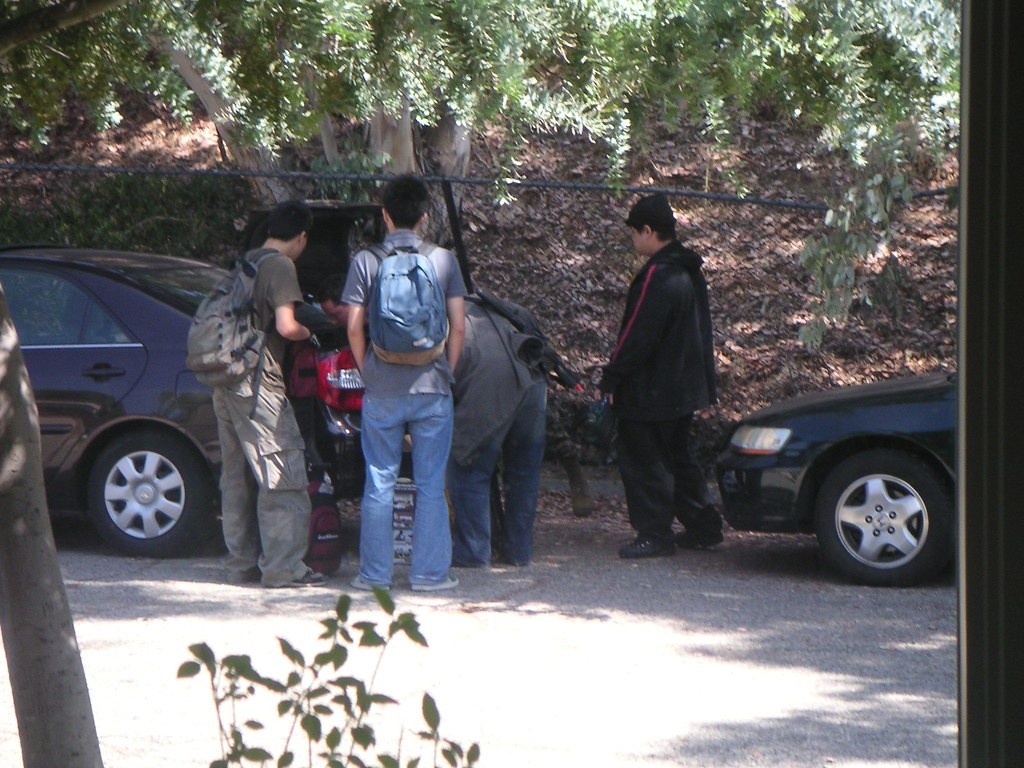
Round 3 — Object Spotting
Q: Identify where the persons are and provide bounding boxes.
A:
[598,191,724,559]
[211,200,328,587]
[341,173,467,593]
[446,283,548,569]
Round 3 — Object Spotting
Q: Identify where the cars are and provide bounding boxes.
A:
[1,198,439,556]
[714,366,961,589]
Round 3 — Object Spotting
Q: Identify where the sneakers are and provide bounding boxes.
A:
[674,528,724,548]
[618,536,675,558]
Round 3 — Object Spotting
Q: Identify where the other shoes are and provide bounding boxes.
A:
[350,576,390,591]
[568,475,593,517]
[282,568,329,587]
[412,575,459,591]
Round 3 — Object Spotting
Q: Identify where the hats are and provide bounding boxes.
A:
[626,193,677,229]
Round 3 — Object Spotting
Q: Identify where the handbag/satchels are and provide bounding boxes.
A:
[571,400,615,446]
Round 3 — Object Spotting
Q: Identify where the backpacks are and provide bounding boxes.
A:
[364,239,450,365]
[185,248,281,387]
[303,504,342,575]
